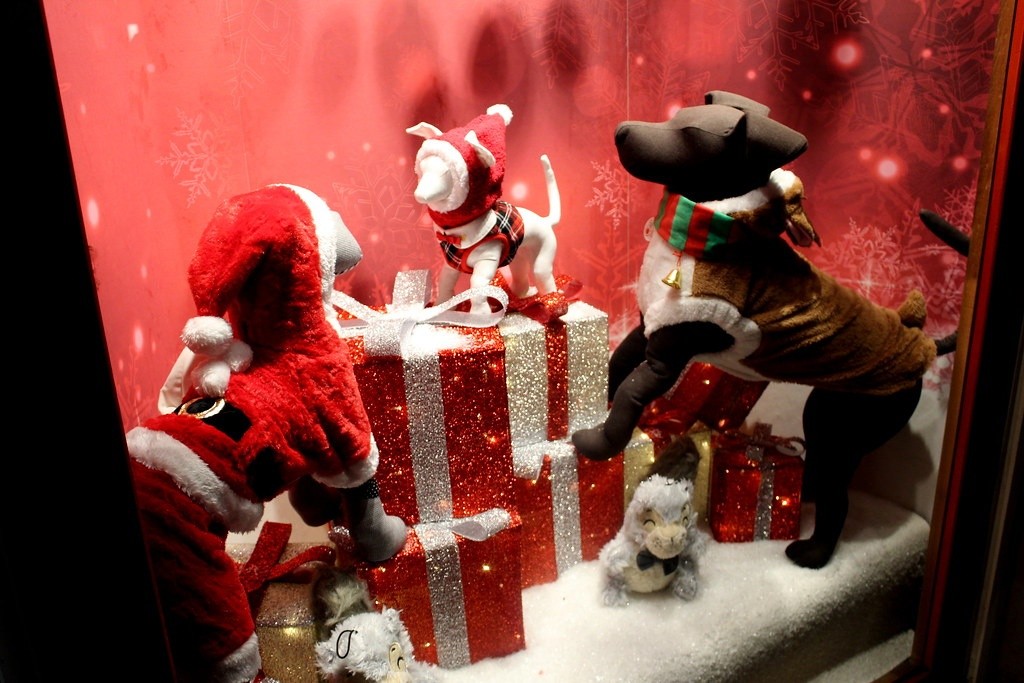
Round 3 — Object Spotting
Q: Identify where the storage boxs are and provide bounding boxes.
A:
[665,352,769,428]
[367,513,527,672]
[707,432,803,543]
[512,405,683,587]
[497,301,608,445]
[331,318,513,517]
